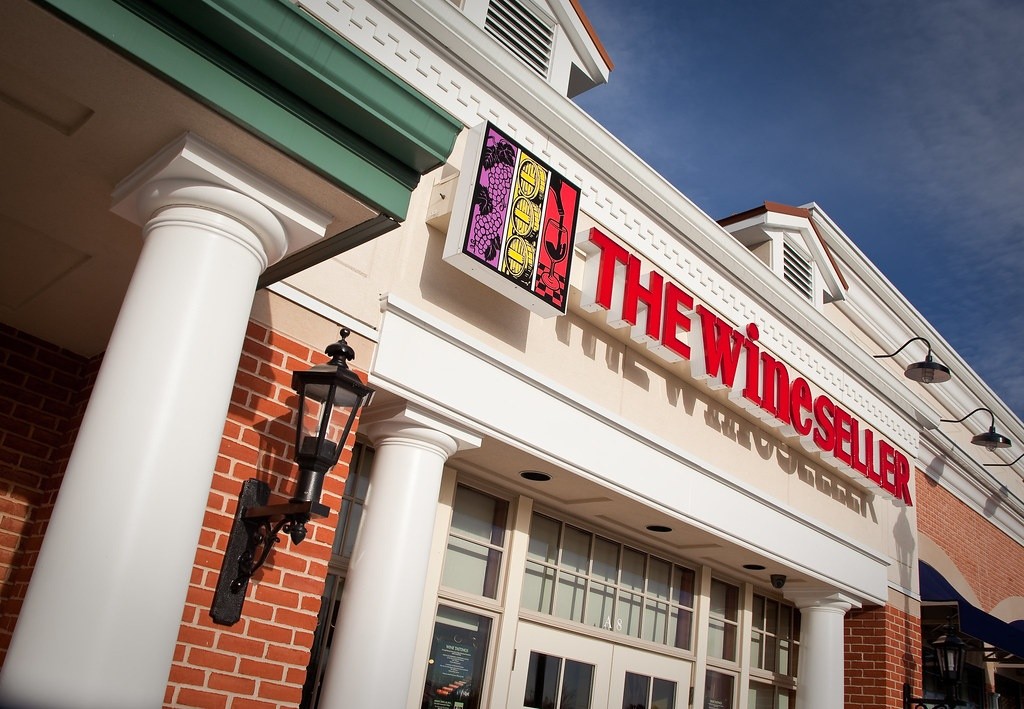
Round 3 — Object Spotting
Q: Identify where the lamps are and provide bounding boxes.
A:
[903,615,967,708]
[873,337,951,384]
[940,407,1012,452]
[209,327,377,627]
